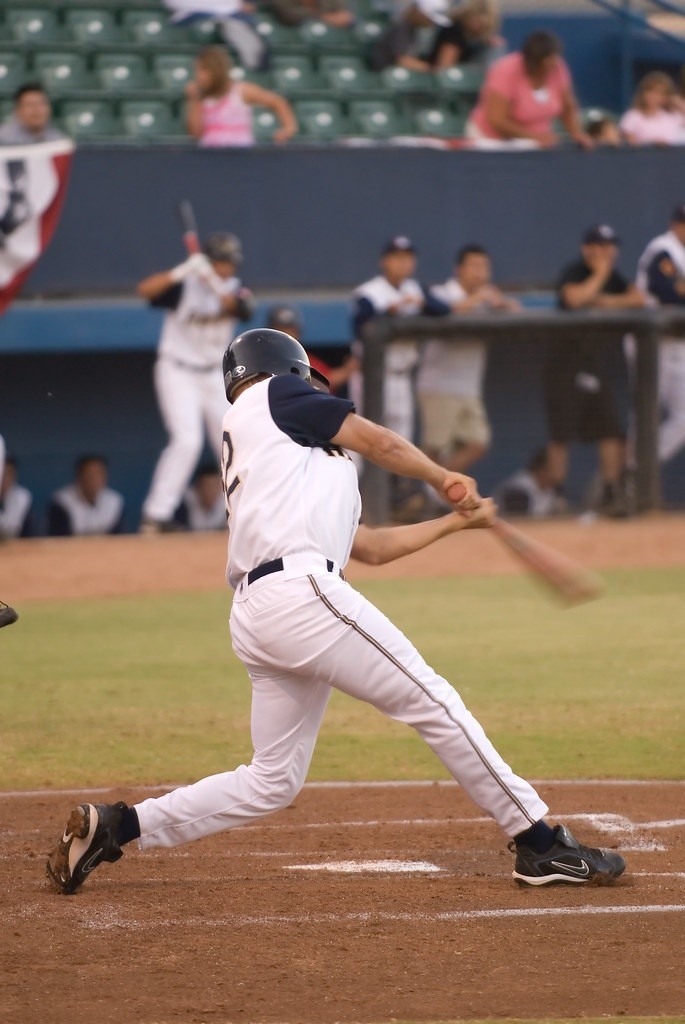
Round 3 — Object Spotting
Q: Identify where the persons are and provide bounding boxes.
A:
[0,464,34,538]
[629,208,685,494]
[138,234,259,532]
[465,31,593,148]
[416,247,520,515]
[0,83,65,142]
[174,467,228,529]
[590,73,685,146]
[392,1,499,75]
[270,305,357,387]
[556,226,643,510]
[47,328,624,893]
[506,445,568,518]
[186,45,297,145]
[165,1,360,30]
[346,237,451,519]
[50,454,127,535]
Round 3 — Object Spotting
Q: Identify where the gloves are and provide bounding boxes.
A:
[168,254,218,288]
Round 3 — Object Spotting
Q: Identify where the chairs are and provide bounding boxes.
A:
[0,0,618,144]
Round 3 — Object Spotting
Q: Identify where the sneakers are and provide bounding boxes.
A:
[44,799,133,895]
[504,820,627,887]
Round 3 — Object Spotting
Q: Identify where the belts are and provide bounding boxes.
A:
[237,555,344,591]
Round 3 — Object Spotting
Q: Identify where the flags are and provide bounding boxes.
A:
[0,144,74,316]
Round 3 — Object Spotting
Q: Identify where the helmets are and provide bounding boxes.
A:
[220,327,332,404]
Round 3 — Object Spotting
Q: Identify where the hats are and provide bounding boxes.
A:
[585,227,619,245]
[380,237,413,254]
[208,233,246,266]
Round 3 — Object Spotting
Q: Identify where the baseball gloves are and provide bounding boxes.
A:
[0,606,19,628]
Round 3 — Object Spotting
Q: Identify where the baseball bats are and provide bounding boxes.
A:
[447,479,610,610]
